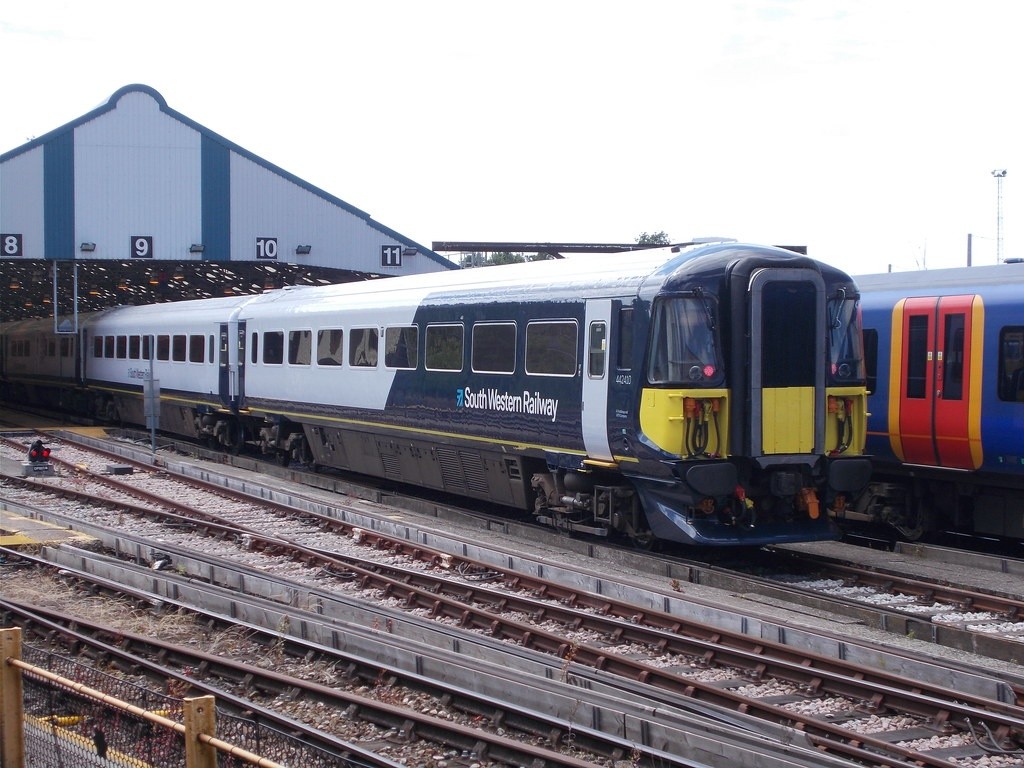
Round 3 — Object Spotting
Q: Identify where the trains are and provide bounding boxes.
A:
[1,241,876,552]
[826,258,1023,550]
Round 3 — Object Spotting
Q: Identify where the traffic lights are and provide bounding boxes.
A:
[29,439,51,462]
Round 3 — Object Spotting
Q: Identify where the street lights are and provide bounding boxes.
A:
[991,168,1006,265]
